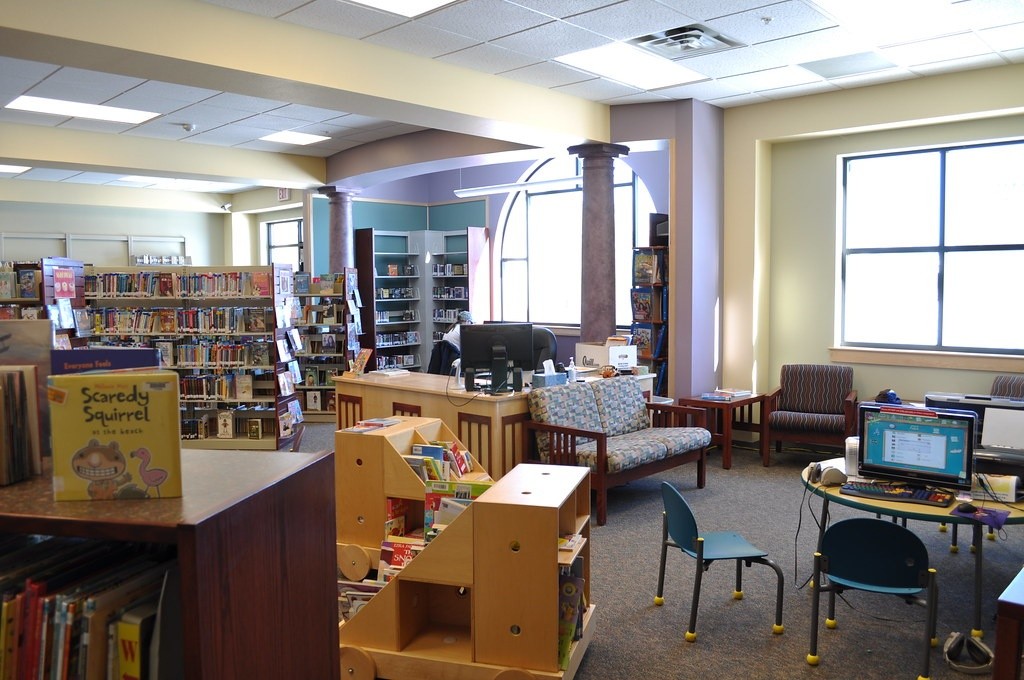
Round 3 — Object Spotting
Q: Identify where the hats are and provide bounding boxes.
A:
[457,311,473,324]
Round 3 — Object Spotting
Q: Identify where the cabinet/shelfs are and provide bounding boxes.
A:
[629,244,671,397]
[1,227,597,680]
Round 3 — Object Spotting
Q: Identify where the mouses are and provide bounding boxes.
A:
[958,504,977,513]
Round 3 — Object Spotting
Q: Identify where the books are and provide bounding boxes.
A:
[631,249,668,398]
[376,262,469,371]
[559,533,592,673]
[0,255,362,501]
[337,418,493,631]
[701,388,752,402]
[0,533,184,680]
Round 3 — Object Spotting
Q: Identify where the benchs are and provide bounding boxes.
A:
[526,376,711,526]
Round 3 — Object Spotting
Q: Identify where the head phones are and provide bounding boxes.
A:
[807,462,847,485]
[944,631,994,674]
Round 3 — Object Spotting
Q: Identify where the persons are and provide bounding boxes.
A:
[428,310,473,374]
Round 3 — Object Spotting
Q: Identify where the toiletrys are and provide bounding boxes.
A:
[568,356,576,383]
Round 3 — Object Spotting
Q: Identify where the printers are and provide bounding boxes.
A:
[924,393,1024,502]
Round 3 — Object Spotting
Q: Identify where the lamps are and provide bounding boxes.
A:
[454,168,583,197]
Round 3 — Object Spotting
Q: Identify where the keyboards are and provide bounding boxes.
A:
[838,481,954,508]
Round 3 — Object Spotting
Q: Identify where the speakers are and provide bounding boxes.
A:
[512,367,523,392]
[464,368,474,391]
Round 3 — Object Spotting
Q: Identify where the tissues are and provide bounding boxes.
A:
[532,359,566,388]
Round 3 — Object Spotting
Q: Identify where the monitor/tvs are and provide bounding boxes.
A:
[857,402,979,493]
[461,323,534,395]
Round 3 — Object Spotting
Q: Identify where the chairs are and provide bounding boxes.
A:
[654,482,784,642]
[763,364,857,465]
[992,374,1024,398]
[807,518,939,680]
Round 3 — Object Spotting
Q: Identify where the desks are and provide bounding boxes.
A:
[677,394,767,469]
[802,457,1024,637]
[332,370,657,484]
[996,566,1024,680]
